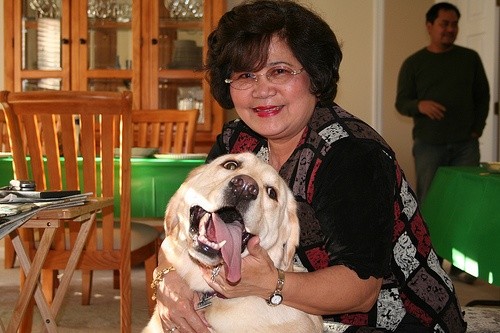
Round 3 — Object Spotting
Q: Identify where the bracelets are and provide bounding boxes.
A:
[150,266,176,301]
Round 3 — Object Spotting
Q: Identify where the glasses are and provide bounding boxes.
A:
[225,62,305,91]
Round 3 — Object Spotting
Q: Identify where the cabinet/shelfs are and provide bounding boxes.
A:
[0,0,228,154]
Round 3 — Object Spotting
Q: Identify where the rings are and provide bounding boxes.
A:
[169,326,178,333]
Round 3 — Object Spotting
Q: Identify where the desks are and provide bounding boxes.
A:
[0,156,205,216]
[418,165,500,292]
[6,196,115,333]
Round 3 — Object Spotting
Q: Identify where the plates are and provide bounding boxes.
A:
[111,146,158,158]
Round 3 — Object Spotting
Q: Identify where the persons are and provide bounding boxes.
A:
[149,1,468,333]
[394,1,490,275]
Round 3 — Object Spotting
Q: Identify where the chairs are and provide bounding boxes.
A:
[0,89,200,333]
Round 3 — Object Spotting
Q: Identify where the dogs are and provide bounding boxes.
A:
[142,150,326,333]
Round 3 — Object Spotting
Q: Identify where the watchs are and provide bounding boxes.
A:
[265,267,286,307]
